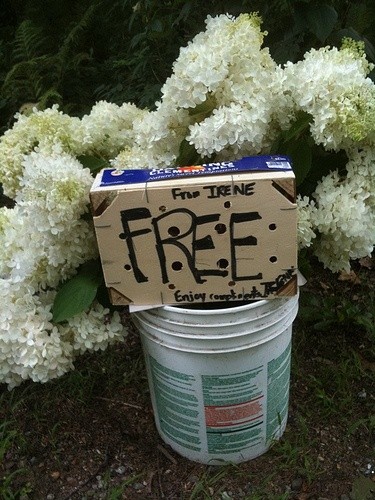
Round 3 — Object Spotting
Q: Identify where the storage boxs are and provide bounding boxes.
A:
[87,155,298,305]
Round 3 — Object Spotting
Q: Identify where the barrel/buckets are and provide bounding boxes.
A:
[132,287,300,466]
